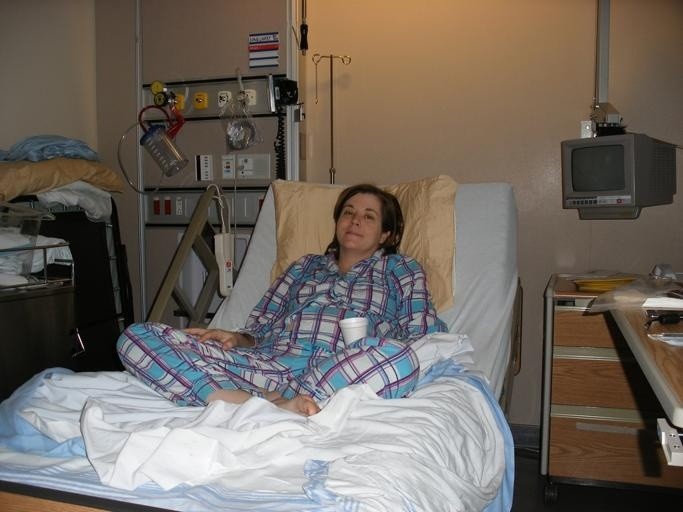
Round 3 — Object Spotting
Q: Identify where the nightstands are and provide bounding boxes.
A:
[540,271,683,501]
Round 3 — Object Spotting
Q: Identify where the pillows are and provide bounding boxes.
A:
[0,158,125,204]
[272,175,458,312]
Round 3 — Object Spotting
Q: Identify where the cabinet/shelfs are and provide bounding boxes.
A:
[136,72,287,327]
[0,243,75,397]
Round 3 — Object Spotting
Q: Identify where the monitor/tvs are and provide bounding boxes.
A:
[561,134,676,221]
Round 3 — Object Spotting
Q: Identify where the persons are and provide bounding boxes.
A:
[116,182,449,418]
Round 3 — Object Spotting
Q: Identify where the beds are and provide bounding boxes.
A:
[0,182,524,512]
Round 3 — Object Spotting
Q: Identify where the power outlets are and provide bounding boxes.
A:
[657,418,683,467]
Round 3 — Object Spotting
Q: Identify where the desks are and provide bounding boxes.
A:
[608,293,683,425]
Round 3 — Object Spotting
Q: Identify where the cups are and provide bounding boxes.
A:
[338,317,369,348]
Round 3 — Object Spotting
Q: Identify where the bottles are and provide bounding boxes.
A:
[140,125,189,177]
